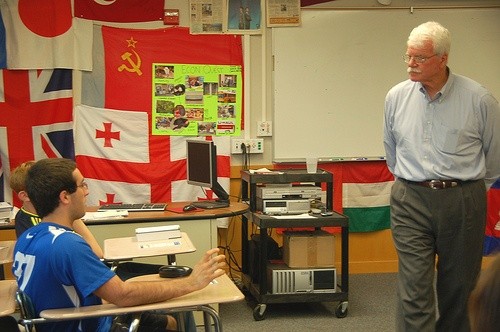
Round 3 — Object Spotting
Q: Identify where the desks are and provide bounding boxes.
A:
[0,198,250,326]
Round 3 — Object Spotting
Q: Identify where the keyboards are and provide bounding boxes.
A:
[98,203,167,211]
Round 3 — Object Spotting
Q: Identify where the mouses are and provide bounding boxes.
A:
[184,205,196,211]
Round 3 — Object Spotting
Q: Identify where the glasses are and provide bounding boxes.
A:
[402,53,437,62]
[76,182,88,190]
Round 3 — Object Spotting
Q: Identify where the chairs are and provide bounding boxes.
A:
[0,232,245,332]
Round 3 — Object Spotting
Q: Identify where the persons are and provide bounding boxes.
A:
[383,20,500,332]
[10,161,104,258]
[11,159,229,332]
[468,255,500,332]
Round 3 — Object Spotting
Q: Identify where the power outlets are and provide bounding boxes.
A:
[231,139,264,154]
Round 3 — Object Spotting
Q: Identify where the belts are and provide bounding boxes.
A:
[401,176,476,189]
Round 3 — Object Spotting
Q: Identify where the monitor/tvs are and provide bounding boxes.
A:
[186,139,230,209]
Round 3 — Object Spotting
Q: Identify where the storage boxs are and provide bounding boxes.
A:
[135,229,182,240]
[281,231,336,268]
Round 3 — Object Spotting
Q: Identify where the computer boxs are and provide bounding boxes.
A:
[262,258,337,294]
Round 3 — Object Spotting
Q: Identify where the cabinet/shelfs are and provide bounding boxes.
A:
[241,170,349,321]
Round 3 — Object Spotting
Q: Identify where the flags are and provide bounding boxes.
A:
[0,0,244,203]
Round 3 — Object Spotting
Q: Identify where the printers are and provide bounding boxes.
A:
[255,186,327,215]
